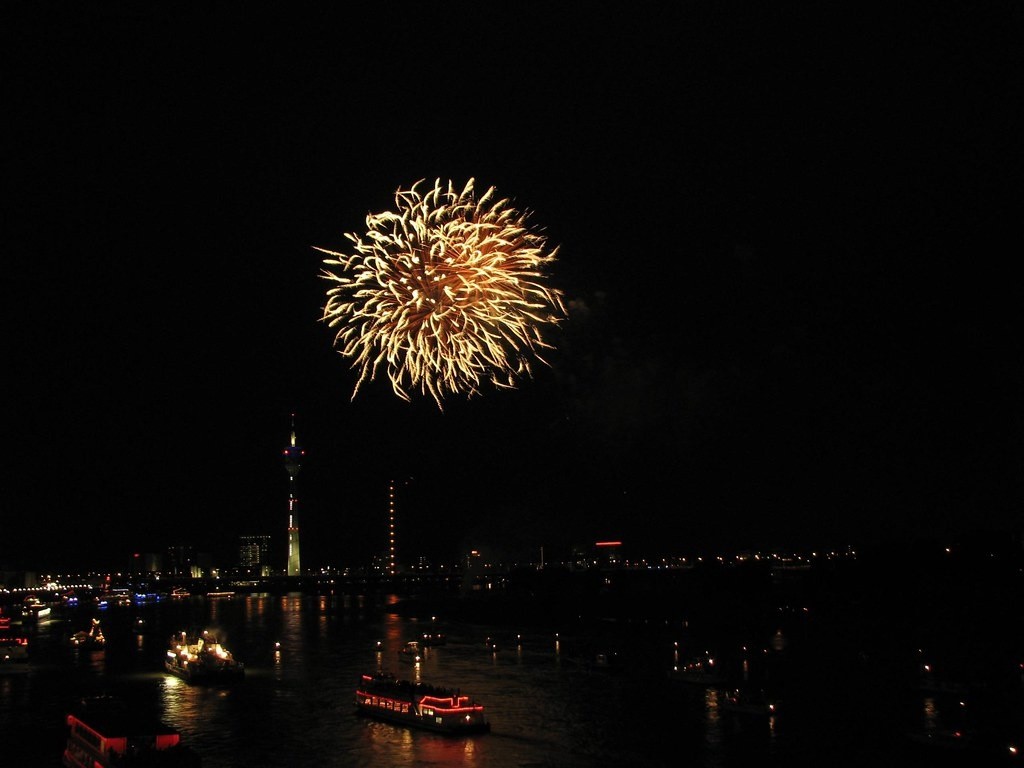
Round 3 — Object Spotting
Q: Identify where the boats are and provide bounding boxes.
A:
[165,630,247,686]
[68,618,109,649]
[0,593,51,663]
[352,670,491,738]
[62,704,203,768]
